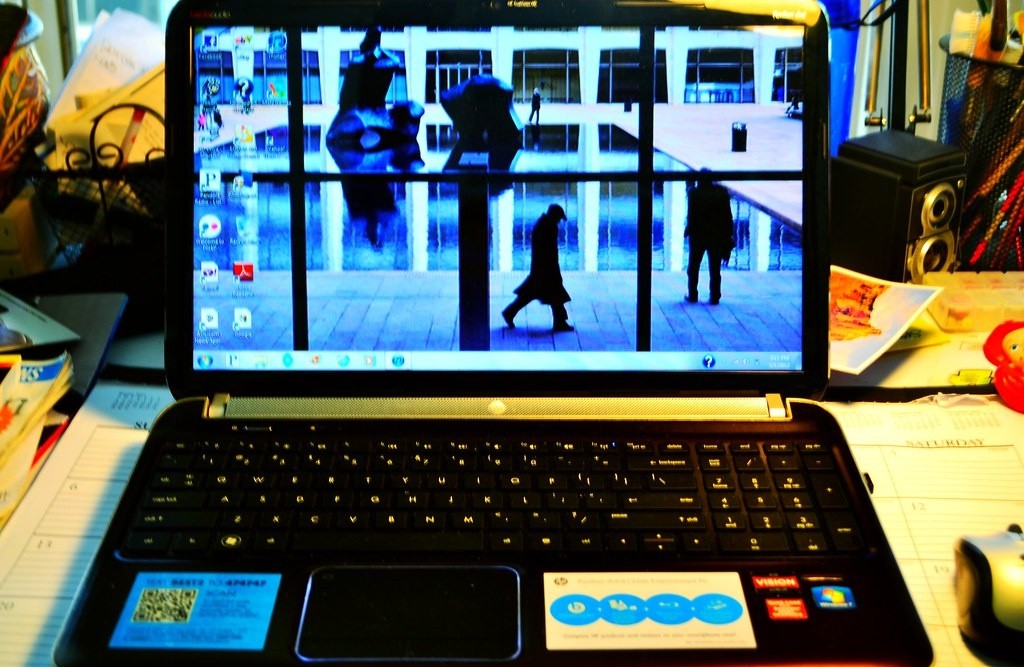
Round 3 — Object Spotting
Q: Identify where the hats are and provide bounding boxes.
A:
[547,203,567,222]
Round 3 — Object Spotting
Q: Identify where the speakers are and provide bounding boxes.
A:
[831,129,966,284]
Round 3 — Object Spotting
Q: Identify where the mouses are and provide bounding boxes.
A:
[954,524,1024,667]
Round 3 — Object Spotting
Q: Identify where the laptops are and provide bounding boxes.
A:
[63,0,934,667]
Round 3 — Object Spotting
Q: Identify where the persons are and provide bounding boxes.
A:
[501,203,576,331]
[682,167,735,305]
[528,87,540,126]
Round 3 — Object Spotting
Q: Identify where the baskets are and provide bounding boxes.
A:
[30,105,166,338]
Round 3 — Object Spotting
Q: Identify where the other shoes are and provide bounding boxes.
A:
[552,322,573,333]
[684,292,697,303]
[501,310,516,329]
[708,297,719,304]
